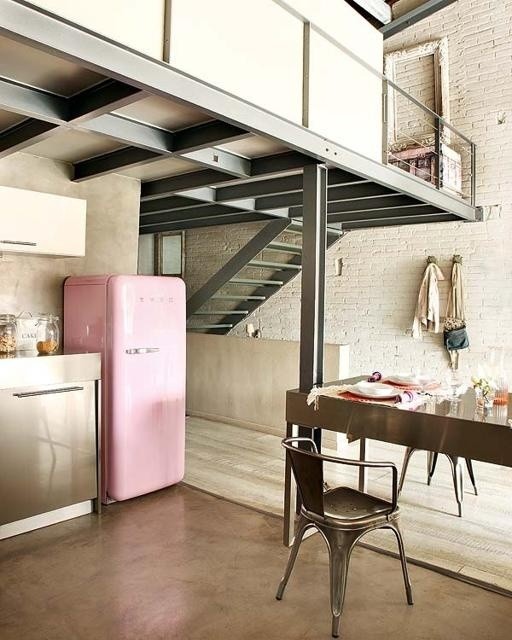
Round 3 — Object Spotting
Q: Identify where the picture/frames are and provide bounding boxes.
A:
[158,230,185,279]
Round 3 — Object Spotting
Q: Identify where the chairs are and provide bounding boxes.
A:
[274,435,415,635]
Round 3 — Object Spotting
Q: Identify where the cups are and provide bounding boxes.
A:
[244,324,255,339]
[423,364,508,413]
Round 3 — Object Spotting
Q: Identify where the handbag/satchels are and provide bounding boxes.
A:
[442,321,471,352]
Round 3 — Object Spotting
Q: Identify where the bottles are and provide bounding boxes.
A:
[365,372,383,382]
[0,312,61,357]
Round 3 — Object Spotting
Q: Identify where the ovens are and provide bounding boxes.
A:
[1,378,104,525]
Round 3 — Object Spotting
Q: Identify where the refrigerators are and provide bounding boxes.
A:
[62,271,187,502]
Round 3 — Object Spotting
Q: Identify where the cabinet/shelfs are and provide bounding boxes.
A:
[388,143,461,195]
[0,183,88,259]
[0,353,101,544]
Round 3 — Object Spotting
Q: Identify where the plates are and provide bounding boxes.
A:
[342,375,426,400]
